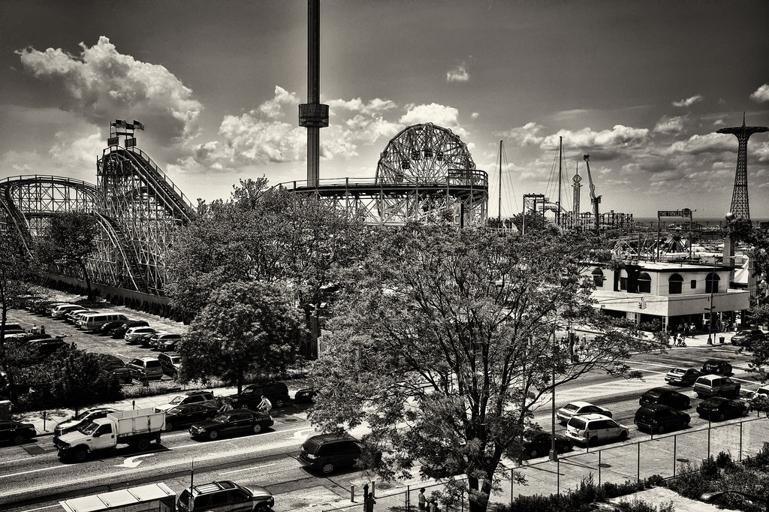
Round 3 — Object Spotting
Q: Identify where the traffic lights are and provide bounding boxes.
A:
[638,301,642,310]
[642,300,647,310]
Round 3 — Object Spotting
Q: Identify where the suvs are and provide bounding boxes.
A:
[297,429,383,474]
[176,479,275,512]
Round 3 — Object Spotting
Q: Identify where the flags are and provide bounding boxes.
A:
[111,119,144,130]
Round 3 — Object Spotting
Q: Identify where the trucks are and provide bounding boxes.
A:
[57,408,167,459]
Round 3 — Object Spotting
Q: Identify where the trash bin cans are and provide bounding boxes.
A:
[720,337,724,343]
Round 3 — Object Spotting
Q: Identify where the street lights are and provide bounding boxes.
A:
[707,256,737,344]
[547,314,564,463]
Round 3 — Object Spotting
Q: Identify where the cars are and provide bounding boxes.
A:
[0,278,195,385]
[156,377,319,454]
[0,418,37,445]
[731,330,765,346]
[53,407,118,435]
[520,311,549,323]
[508,356,769,458]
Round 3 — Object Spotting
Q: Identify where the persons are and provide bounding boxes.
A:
[216,400,233,412]
[418,488,428,510]
[366,492,376,512]
[257,394,272,412]
